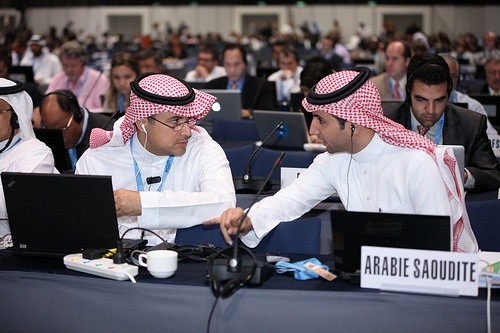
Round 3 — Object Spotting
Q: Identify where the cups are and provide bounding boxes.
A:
[138,250,178,278]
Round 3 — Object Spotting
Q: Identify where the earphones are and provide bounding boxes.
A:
[351,124,356,132]
[141,124,147,134]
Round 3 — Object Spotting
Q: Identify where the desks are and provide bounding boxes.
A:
[0,42,500,333]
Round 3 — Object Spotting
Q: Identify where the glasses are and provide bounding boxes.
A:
[198,58,213,62]
[60,113,74,132]
[150,116,196,131]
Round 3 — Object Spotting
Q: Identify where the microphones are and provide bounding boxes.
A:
[73,108,121,171]
[208,151,286,287]
[233,121,284,194]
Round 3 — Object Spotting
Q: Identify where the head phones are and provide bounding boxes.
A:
[39,91,84,124]
[405,64,454,107]
[219,42,252,67]
[9,113,20,130]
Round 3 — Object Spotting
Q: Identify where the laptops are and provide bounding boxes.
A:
[438,145,468,200]
[0,172,148,257]
[329,209,452,274]
[253,109,317,149]
[197,89,242,124]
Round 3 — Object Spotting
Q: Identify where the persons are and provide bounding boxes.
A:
[71,70,238,247]
[0,11,500,200]
[199,67,481,260]
[0,75,64,243]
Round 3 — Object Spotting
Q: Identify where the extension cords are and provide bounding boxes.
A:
[63,253,139,282]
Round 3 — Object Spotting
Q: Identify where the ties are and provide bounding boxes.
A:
[417,125,430,136]
[231,82,238,89]
[393,82,401,98]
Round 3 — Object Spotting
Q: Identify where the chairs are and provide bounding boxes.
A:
[214,118,260,141]
[175,223,235,249]
[464,199,500,252]
[252,147,317,181]
[253,218,321,255]
[224,145,254,176]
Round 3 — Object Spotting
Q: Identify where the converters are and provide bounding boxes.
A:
[145,242,179,253]
